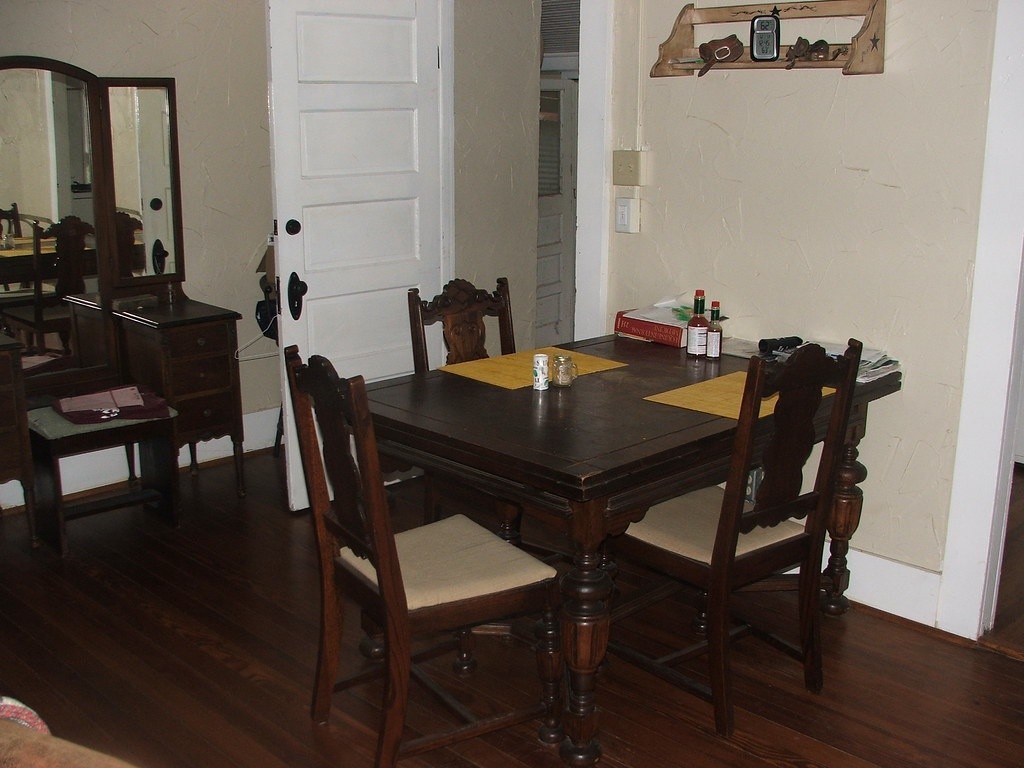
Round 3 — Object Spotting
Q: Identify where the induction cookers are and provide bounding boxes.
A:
[71,176,91,192]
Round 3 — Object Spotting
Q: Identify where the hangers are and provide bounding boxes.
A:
[233,315,280,360]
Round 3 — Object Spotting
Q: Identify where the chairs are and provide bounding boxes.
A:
[284,277,870,768]
[2,215,97,351]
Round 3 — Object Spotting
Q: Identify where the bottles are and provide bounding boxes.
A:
[4,234,15,250]
[687,290,709,357]
[707,301,722,360]
[167,282,176,303]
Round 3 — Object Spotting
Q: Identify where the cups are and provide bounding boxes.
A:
[553,356,578,388]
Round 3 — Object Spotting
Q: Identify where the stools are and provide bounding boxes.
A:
[31,392,180,565]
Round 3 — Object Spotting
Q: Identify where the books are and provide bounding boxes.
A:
[773,339,900,385]
[614,301,712,348]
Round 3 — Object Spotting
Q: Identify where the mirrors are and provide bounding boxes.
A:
[0,54,120,382]
[100,77,187,282]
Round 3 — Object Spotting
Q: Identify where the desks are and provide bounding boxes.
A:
[0,234,98,291]
[349,332,905,768]
[0,272,96,309]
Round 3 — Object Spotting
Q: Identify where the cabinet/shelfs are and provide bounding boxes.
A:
[123,298,249,507]
[62,288,109,366]
[0,334,41,547]
